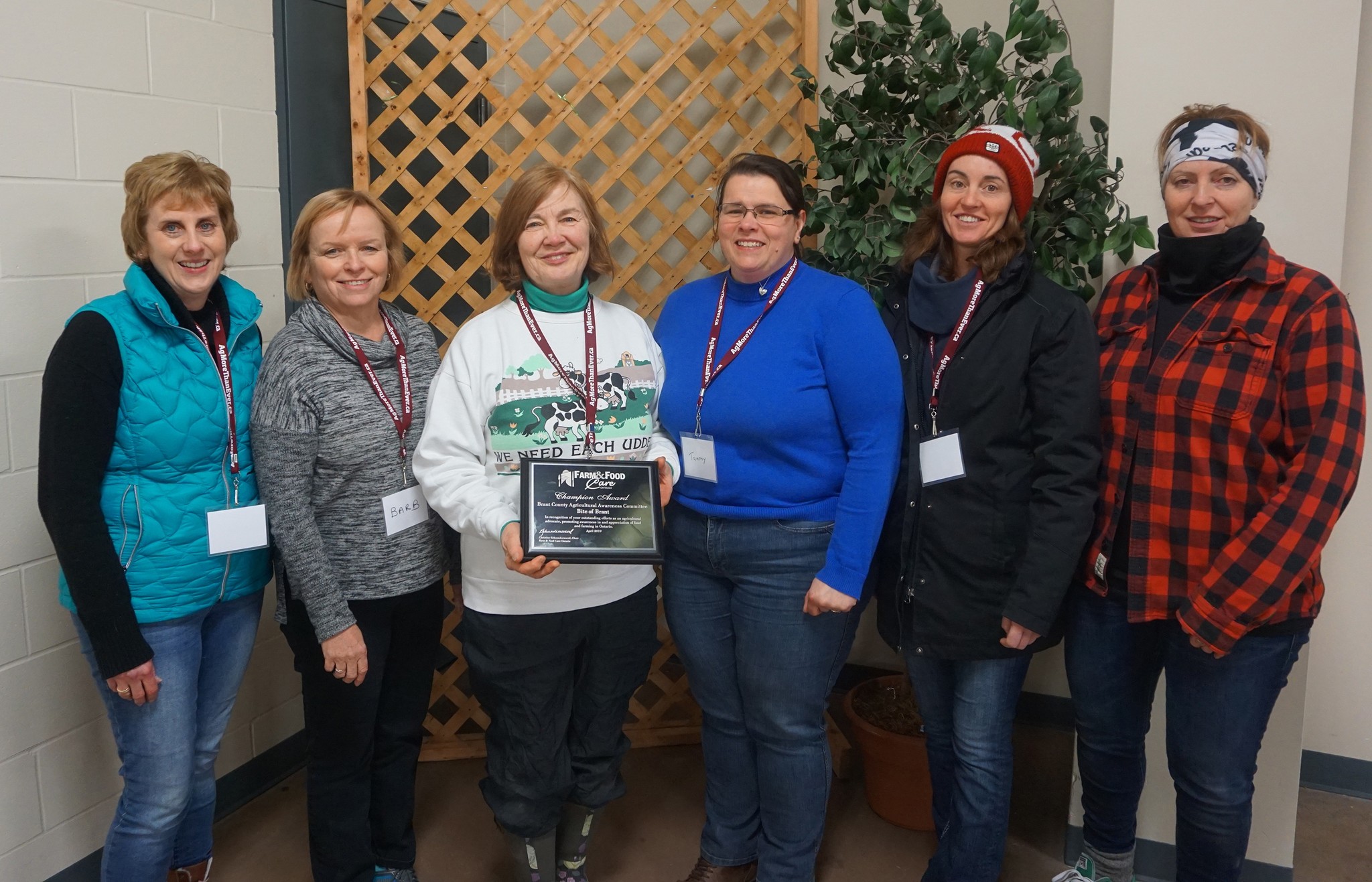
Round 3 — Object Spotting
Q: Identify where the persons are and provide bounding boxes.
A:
[38,154,276,882]
[248,187,462,882]
[651,153,906,882]
[411,164,681,882]
[1049,104,1366,882]
[877,123,1102,882]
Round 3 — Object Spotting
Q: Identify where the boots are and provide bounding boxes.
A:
[176,856,214,882]
[492,817,557,881]
[554,802,594,882]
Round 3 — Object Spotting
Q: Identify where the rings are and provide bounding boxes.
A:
[335,668,345,673]
[831,610,840,613]
[116,686,130,694]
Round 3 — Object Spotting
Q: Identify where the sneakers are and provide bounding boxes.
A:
[371,864,418,882]
[1051,851,1136,882]
[680,859,753,882]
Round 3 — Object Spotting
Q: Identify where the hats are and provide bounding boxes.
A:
[932,124,1040,223]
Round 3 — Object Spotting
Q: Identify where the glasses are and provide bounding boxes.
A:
[718,203,797,226]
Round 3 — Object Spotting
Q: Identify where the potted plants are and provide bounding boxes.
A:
[790,0,1152,830]
